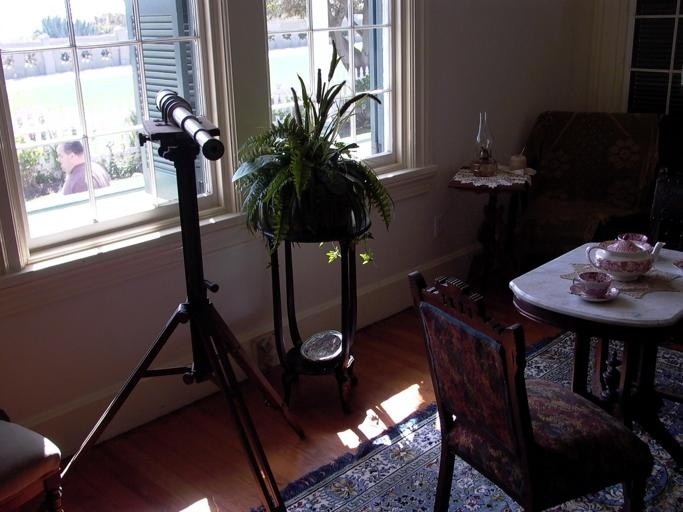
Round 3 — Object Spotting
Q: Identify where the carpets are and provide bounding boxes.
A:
[251,330,683,512]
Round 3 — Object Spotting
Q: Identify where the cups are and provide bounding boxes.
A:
[573,272,612,298]
[617,232,648,243]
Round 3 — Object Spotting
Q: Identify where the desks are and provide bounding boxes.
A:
[448,163,538,290]
[262,214,372,415]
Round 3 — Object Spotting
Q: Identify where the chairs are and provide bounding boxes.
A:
[508,112,661,267]
[409,270,653,512]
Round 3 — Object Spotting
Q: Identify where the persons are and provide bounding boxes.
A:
[55,139,111,194]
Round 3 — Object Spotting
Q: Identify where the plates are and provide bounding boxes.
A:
[570,285,619,304]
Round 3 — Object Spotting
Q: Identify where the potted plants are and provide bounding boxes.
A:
[234,40,396,254]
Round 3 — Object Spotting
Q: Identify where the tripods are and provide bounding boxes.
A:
[60,115,306,512]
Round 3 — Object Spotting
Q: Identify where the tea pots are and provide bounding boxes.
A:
[586,233,665,282]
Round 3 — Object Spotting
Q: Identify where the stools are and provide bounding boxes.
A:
[0,408,64,512]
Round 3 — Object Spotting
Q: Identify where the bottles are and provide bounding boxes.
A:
[475,111,496,177]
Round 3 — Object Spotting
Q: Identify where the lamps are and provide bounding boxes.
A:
[471,112,497,177]
